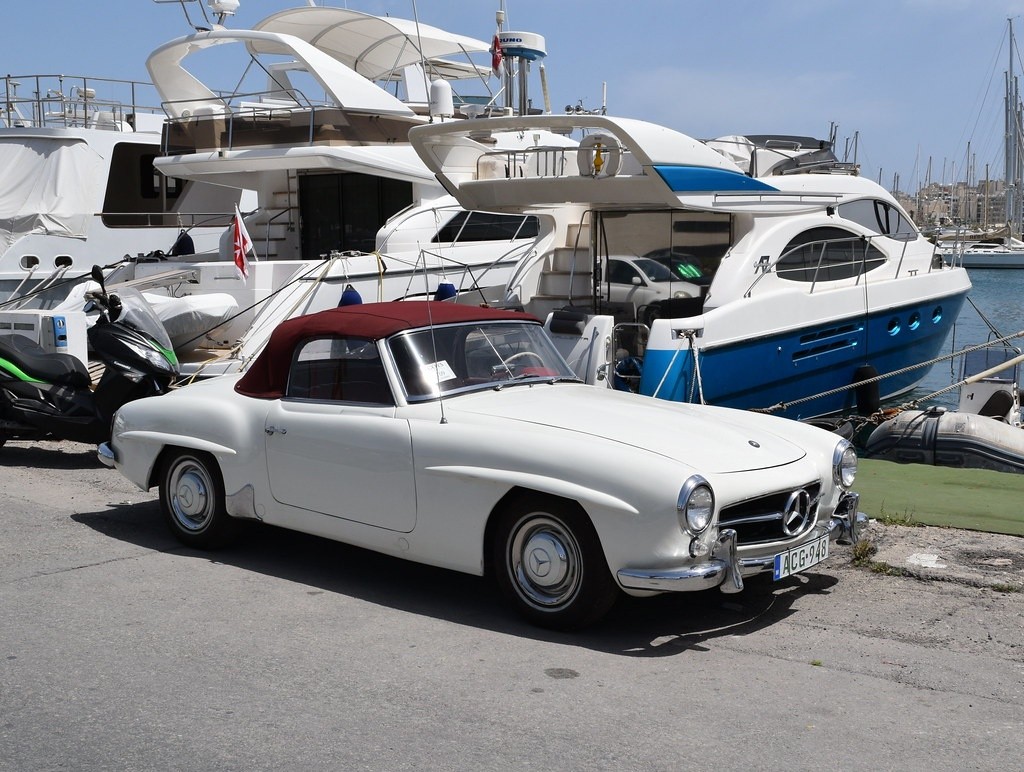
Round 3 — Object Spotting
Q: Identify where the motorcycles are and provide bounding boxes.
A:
[0,265,181,448]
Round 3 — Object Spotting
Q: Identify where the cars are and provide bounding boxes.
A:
[98,297,868,632]
[597,255,701,310]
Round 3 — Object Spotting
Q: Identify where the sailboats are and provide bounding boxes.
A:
[876,17,1024,269]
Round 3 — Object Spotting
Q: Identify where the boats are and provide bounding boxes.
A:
[867,328,1024,476]
[0,0,589,379]
[246,41,973,433]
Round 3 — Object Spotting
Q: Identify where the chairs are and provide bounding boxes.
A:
[279,358,385,407]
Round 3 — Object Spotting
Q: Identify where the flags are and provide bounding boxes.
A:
[491,26,503,79]
[234,206,253,286]
